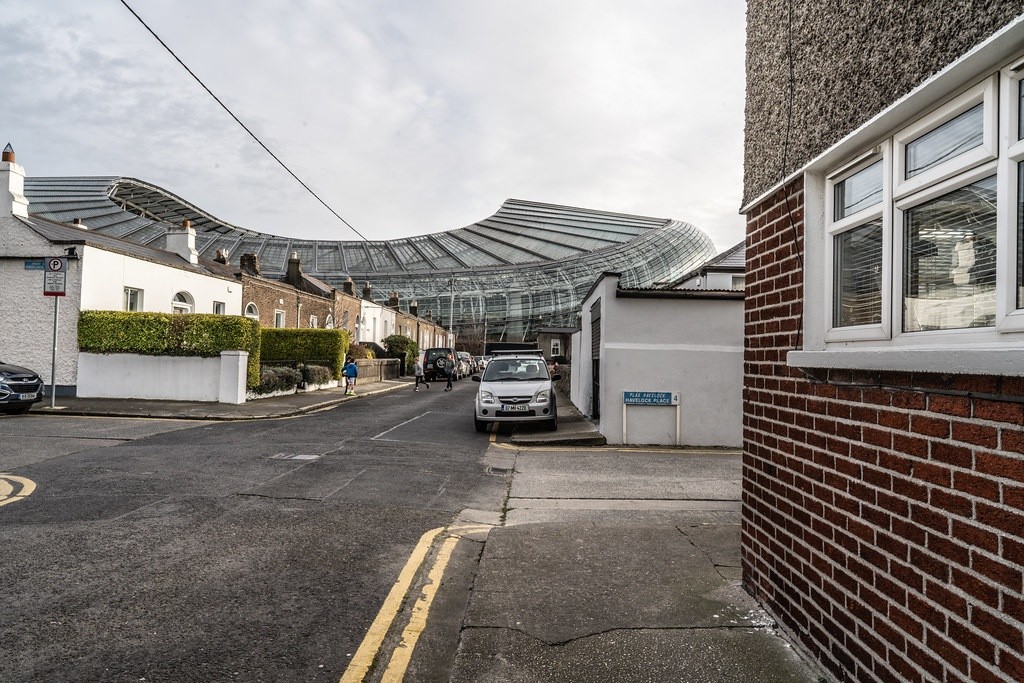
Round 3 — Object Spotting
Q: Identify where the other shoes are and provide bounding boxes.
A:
[346,392,350,395]
[445,388,448,391]
[414,387,419,392]
[449,388,453,391]
[426,382,430,388]
[350,393,355,395]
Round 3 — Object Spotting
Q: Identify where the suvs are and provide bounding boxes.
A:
[422,347,464,382]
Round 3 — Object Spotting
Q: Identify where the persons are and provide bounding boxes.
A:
[414,357,430,392]
[444,353,456,391]
[341,356,360,396]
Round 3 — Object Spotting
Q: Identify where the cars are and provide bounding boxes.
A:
[471,349,561,432]
[456,350,494,378]
[0,360,45,415]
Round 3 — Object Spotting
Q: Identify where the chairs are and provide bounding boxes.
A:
[497,362,512,376]
[526,365,539,377]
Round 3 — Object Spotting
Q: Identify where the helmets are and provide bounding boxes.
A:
[343,370,347,375]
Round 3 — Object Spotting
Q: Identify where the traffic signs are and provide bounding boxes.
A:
[43,257,67,296]
